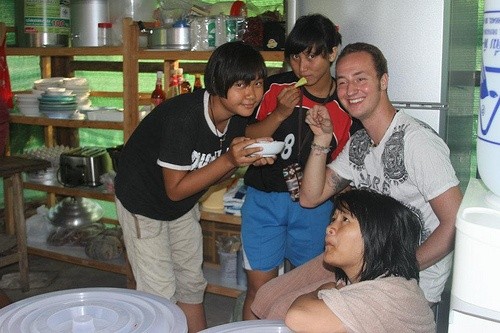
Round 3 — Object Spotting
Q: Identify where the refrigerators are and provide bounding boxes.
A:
[287,0,476,333]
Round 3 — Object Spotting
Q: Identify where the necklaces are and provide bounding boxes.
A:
[209,94,228,156]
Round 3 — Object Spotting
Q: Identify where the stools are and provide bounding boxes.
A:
[0,156,50,293]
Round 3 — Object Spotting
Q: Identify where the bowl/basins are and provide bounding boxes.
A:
[15,76,90,120]
[243,141,285,157]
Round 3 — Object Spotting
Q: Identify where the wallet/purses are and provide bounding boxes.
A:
[282,162,304,202]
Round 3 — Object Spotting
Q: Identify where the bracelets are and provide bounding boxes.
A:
[310,143,332,154]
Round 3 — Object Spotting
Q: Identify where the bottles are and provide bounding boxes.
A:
[150,67,202,112]
[97,22,112,47]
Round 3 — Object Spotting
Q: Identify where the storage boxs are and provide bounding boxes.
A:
[0,288,294,333]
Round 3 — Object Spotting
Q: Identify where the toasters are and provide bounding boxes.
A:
[56,146,112,188]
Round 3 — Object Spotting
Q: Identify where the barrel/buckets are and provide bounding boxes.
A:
[0,288,189,333]
[196,319,296,333]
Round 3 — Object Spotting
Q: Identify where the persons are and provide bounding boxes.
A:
[113,41,277,333]
[283,187,437,333]
[299,42,464,314]
[223,13,363,321]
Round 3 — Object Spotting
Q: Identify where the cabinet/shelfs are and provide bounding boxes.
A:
[0,17,285,299]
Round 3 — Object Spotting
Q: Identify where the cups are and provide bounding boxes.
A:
[219,252,237,284]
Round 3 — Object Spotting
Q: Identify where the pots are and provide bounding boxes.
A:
[137,21,194,50]
